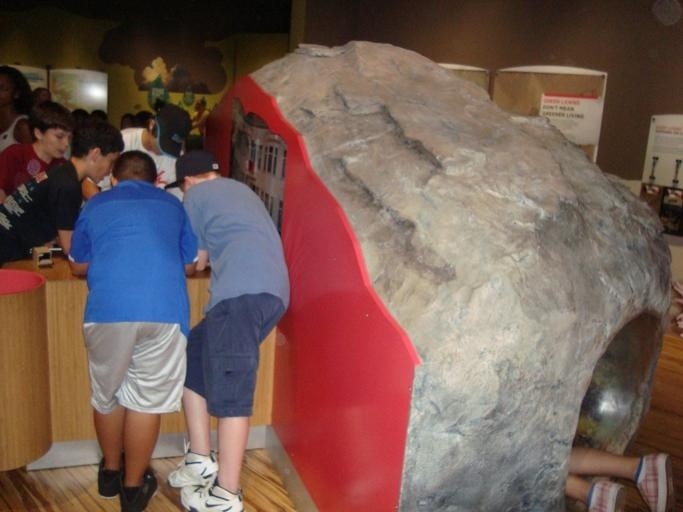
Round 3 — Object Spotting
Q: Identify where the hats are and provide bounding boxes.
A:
[163,148,221,190]
[153,102,192,159]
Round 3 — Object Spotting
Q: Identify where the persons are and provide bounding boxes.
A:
[159,150,290,512]
[566,446,675,510]
[70,150,198,511]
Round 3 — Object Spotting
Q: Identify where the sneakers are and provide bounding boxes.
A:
[178,482,245,512]
[97,451,125,500]
[635,450,680,512]
[167,448,219,488]
[118,465,158,512]
[585,478,627,512]
